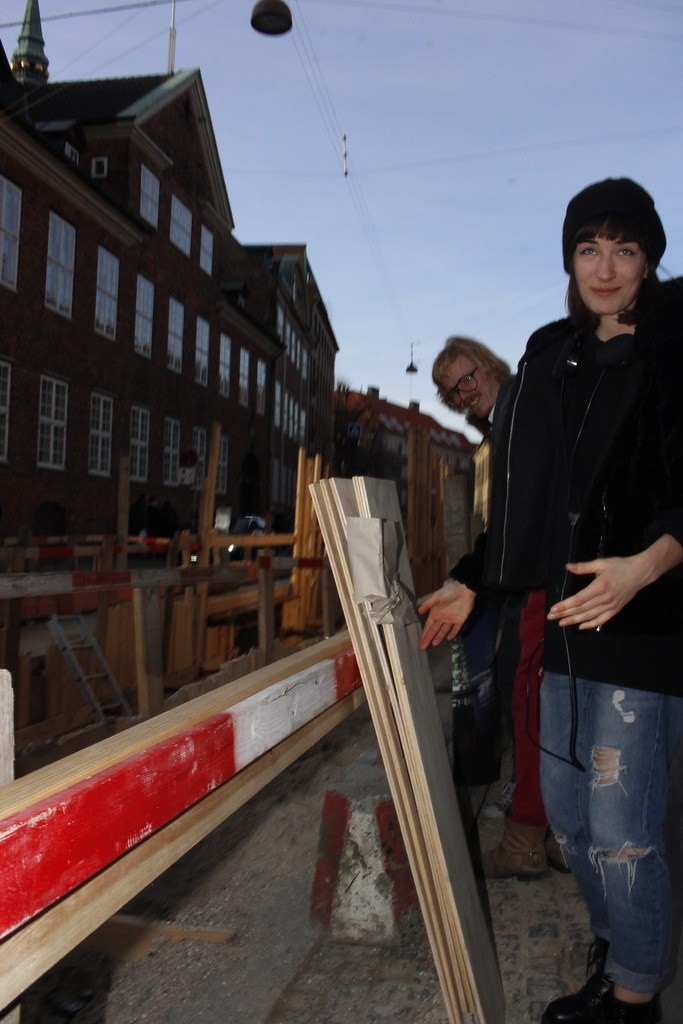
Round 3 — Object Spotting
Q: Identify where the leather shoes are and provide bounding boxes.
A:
[540,936,662,1024]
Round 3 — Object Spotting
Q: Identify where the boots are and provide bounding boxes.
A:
[477,802,547,881]
[544,828,572,873]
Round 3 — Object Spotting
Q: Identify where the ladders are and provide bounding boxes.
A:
[46,613,134,728]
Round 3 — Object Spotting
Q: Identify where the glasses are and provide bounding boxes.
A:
[444,366,479,408]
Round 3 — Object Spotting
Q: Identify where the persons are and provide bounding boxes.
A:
[418,177,683,1024]
[430,336,571,878]
[129,494,181,561]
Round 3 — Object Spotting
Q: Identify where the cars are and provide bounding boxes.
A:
[176,515,281,565]
